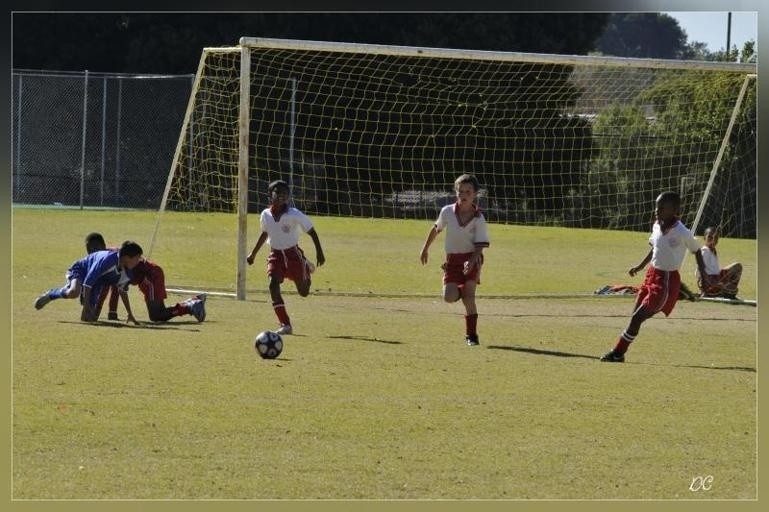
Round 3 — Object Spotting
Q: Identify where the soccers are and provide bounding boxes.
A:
[254,330,285,359]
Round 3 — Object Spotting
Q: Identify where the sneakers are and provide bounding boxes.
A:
[465,336,479,347]
[183,292,208,323]
[600,352,626,363]
[35,289,54,310]
[272,323,294,336]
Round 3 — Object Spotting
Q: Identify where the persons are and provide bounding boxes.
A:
[599,190,712,364]
[83,231,207,325]
[33,238,142,328]
[420,171,490,347]
[245,177,325,337]
[694,224,743,301]
